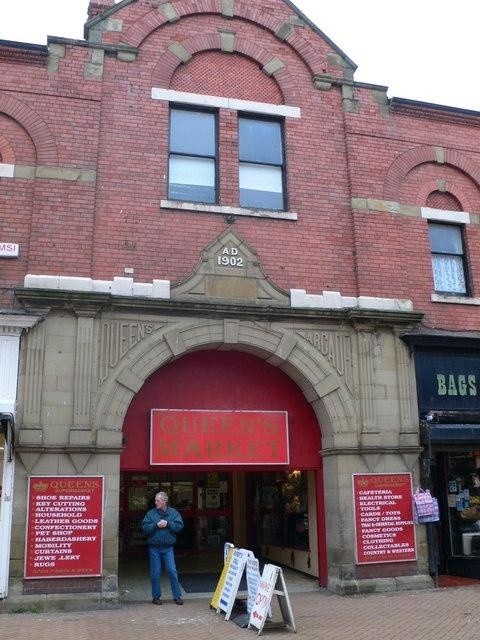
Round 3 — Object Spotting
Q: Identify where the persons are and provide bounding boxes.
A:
[141,491,185,605]
